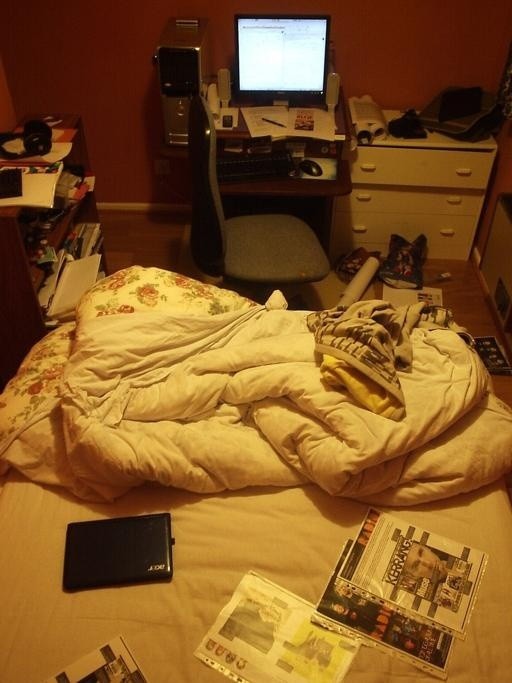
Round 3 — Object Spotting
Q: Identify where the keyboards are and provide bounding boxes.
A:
[216,150,296,185]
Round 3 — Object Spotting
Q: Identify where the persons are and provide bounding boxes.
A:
[402,540,447,584]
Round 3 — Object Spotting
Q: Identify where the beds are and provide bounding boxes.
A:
[1,303,508,683]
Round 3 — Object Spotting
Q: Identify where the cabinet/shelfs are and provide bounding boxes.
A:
[330,112,498,265]
[1,116,107,391]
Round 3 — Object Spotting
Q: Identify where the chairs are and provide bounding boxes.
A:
[186,96,332,285]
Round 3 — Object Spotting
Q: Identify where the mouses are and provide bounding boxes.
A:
[299,159,323,176]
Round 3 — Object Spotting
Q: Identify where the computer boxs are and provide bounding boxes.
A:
[156,16,211,147]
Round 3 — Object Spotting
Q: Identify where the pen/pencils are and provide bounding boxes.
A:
[262,117,286,127]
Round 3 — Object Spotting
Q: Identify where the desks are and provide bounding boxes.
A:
[203,98,350,240]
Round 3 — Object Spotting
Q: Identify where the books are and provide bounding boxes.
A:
[471,335,512,376]
[193,569,361,683]
[1,124,106,327]
[334,507,489,640]
[311,538,454,681]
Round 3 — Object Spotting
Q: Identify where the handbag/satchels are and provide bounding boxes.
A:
[417,85,503,142]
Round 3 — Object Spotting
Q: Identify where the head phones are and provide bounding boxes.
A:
[0,119,52,160]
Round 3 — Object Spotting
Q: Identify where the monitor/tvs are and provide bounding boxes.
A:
[231,13,331,107]
